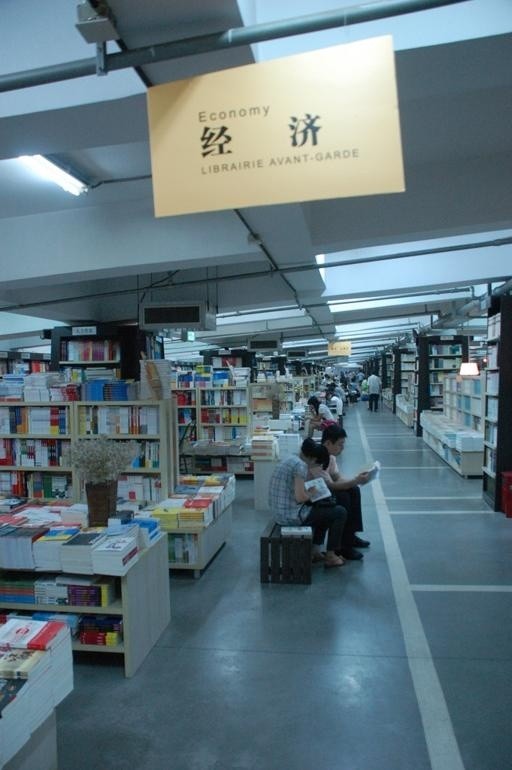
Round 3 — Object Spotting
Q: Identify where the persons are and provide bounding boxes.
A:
[311,425,370,560]
[300,369,383,440]
[268,438,346,568]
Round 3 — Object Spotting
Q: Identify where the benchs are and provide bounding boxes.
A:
[256,518,320,587]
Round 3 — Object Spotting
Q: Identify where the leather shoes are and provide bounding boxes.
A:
[331,546,364,560]
[350,535,370,547]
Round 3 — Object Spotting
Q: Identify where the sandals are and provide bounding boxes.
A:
[311,552,326,564]
[323,556,346,566]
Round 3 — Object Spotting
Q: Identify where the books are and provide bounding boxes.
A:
[370,312,501,502]
[1,574,123,769]
[362,460,381,487]
[0,339,330,568]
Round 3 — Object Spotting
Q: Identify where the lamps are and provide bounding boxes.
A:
[17,152,90,198]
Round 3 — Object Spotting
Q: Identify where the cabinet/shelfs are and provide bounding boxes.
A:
[358,281,511,514]
[153,346,334,511]
[75,396,180,511]
[2,618,76,766]
[0,399,74,505]
[152,472,239,579]
[3,499,173,677]
